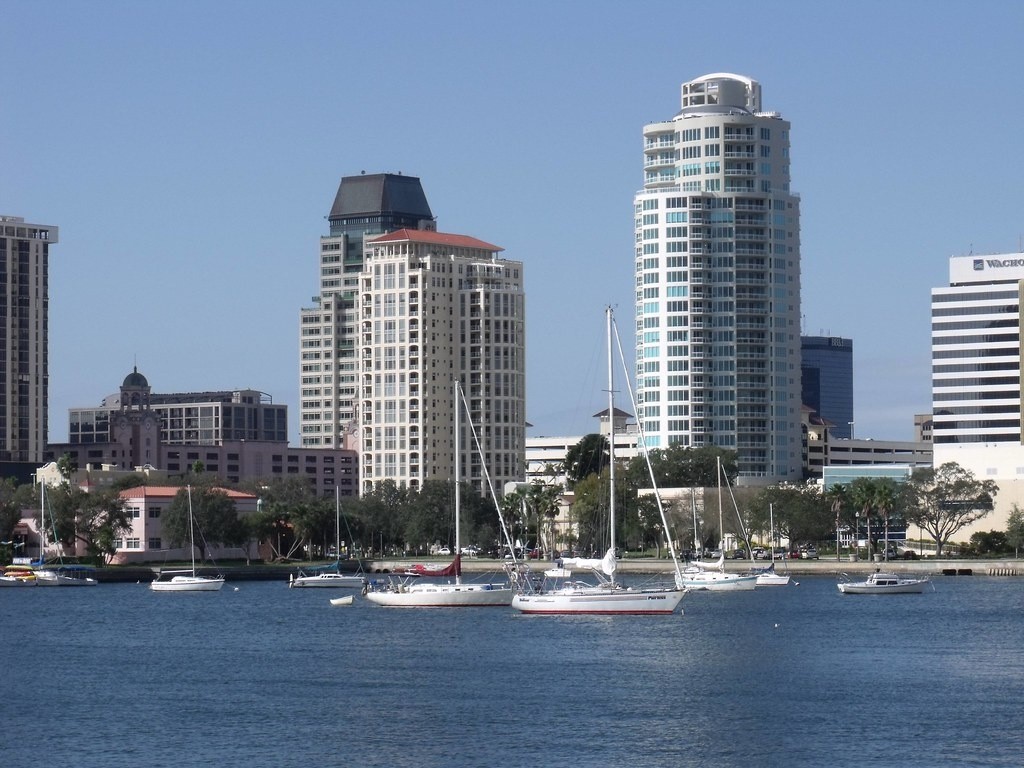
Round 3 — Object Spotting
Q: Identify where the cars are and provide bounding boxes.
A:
[679,543,820,561]
[904,550,916,560]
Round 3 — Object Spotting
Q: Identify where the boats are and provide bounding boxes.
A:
[836,565,930,594]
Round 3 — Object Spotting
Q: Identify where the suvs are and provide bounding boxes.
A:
[883,547,897,560]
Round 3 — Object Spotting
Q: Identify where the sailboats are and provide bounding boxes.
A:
[289,486,367,587]
[754,502,790,586]
[150,485,225,591]
[511,303,689,614]
[674,455,759,591]
[0,478,98,586]
[368,380,523,606]
